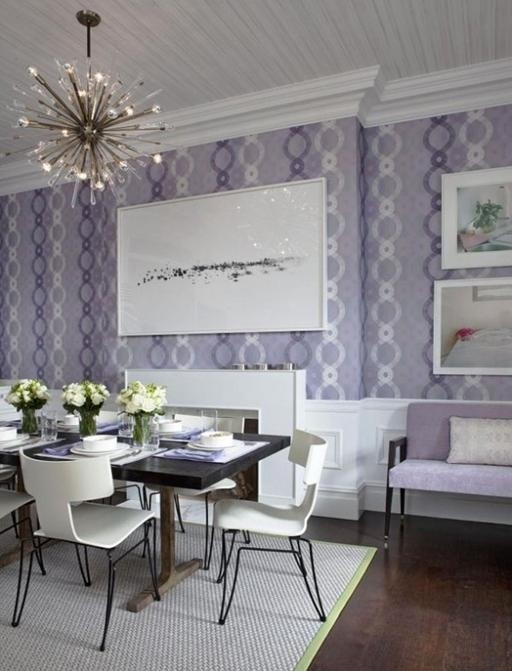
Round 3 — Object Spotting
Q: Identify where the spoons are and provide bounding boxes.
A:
[110,448,142,463]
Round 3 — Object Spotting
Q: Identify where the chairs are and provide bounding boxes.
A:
[384,402,512,540]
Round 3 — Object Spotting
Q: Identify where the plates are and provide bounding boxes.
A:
[58,423,80,425]
[69,443,129,457]
[187,439,244,451]
[0,433,30,444]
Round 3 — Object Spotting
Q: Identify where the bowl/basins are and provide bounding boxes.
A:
[65,414,98,424]
[200,430,233,446]
[83,434,117,450]
[0,427,17,441]
[159,419,184,432]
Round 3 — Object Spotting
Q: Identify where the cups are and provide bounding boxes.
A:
[117,407,136,438]
[40,409,58,441]
[202,410,217,431]
[141,415,160,452]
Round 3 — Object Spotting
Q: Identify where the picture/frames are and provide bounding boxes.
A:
[117,177,328,339]
[432,278,512,377]
[441,167,512,272]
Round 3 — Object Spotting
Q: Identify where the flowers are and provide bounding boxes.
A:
[116,382,167,445]
[4,378,49,432]
[62,380,111,434]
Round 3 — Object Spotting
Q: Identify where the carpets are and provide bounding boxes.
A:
[0,498,379,671]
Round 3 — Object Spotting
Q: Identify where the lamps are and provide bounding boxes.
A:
[6,11,175,208]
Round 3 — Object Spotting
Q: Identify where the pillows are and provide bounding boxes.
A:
[446,416,512,466]
